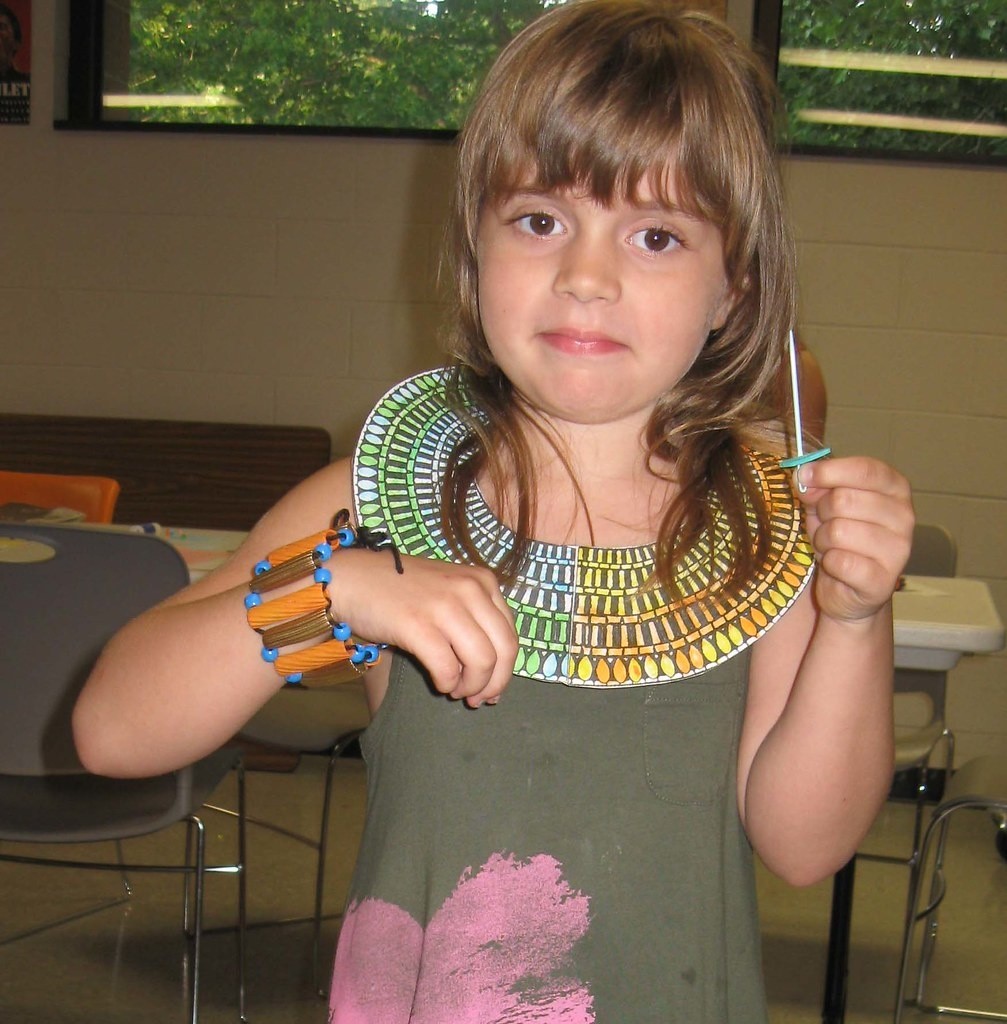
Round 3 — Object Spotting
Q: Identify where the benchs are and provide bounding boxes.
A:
[0,413,331,532]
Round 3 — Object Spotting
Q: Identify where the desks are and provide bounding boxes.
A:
[75,522,1004,1024]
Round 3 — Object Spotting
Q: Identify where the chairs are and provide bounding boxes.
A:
[0,472,1007,1024]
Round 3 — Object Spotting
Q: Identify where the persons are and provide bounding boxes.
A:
[71,3,915,1024]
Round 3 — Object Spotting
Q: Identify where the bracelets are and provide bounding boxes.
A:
[244,510,404,691]
